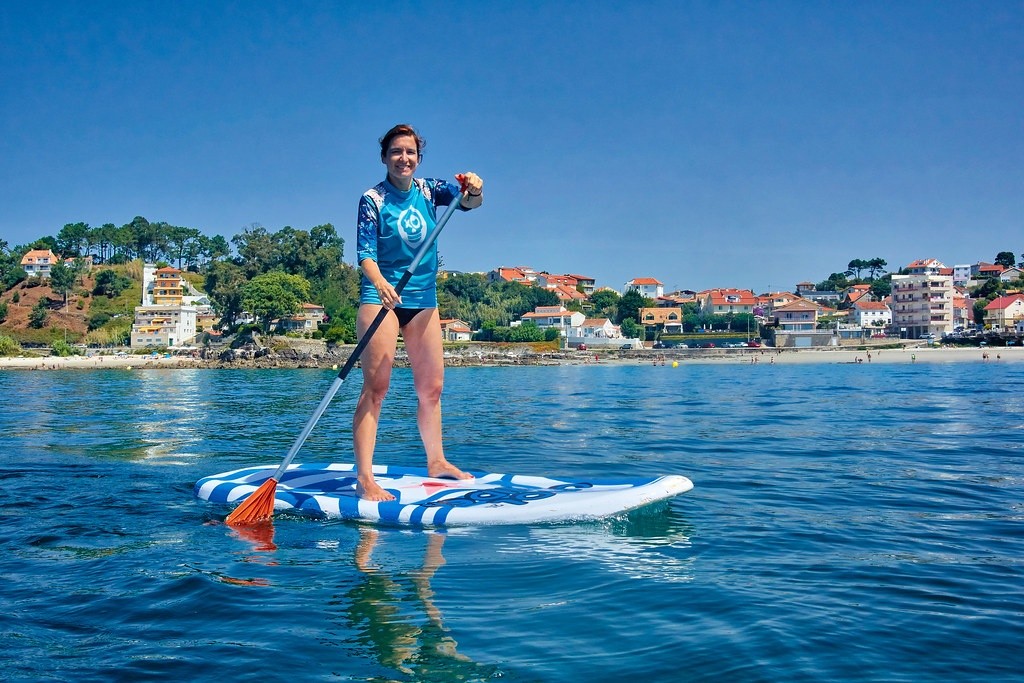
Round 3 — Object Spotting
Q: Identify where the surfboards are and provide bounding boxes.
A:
[195,458,692,529]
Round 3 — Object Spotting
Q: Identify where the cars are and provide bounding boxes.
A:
[577,343,587,350]
[653,340,761,349]
[619,344,633,349]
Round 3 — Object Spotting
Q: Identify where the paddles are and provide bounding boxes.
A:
[222,172,469,527]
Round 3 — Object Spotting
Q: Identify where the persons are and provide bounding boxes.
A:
[353,124,484,501]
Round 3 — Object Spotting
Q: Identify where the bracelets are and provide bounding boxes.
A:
[467,190,482,201]
